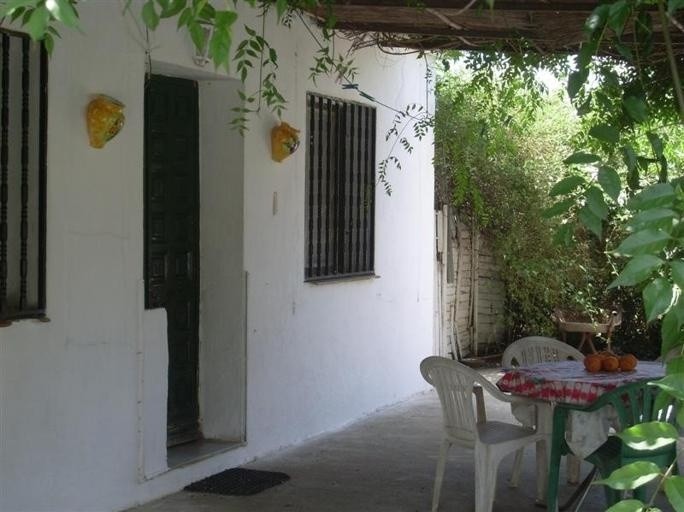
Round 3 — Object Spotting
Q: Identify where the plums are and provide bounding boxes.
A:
[583,353,637,372]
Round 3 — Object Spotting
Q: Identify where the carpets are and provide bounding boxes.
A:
[184,466,290,496]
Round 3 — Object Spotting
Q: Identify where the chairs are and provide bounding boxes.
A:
[500,336,587,487]
[544,375,676,511]
[419,356,550,511]
